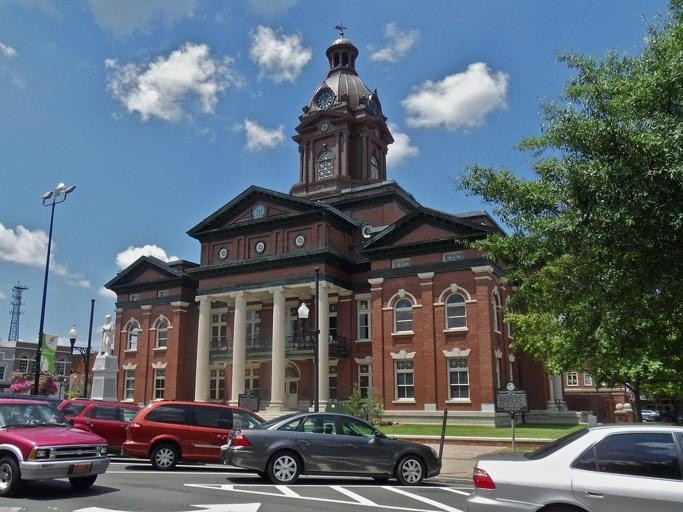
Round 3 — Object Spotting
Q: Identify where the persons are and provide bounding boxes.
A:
[99,314,114,357]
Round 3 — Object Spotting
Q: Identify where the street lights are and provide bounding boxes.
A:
[29,182,76,393]
[69,299,94,400]
[296,265,320,414]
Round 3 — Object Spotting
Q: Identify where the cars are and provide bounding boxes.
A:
[641,410,661,422]
[218,412,441,483]
[0,394,267,496]
[463,409,683,512]
